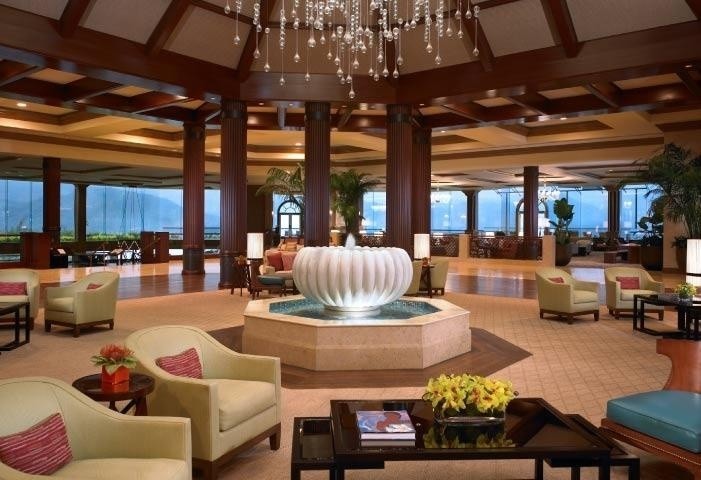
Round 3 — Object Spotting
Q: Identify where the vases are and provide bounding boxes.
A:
[433,406,507,427]
[100,366,129,392]
[677,294,693,299]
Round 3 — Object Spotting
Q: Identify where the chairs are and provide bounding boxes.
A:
[0,268,120,338]
[597,335,701,480]
[247,232,449,298]
[535,267,665,324]
[0,376,192,480]
[126,326,282,480]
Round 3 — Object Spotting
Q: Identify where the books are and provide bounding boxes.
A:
[359,440,418,447]
[355,409,417,441]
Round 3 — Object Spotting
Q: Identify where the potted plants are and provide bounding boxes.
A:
[549,198,575,266]
[640,236,662,270]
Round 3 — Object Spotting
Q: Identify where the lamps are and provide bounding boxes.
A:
[538,177,560,203]
[225,0,480,100]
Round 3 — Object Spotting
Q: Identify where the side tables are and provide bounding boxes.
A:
[71,374,155,416]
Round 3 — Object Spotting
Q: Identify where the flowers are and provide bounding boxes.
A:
[423,375,517,411]
[423,427,514,448]
[674,282,696,298]
[91,344,138,374]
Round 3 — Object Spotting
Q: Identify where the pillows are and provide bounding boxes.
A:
[0,413,73,475]
[159,349,202,379]
